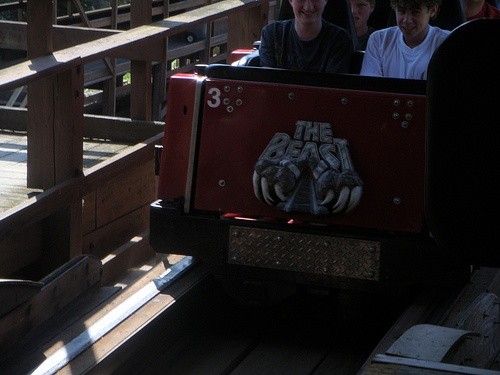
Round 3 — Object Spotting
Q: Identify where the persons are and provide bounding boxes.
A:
[348,0,380,51]
[356,0,453,80]
[458,0,500,27]
[259,0,355,74]
